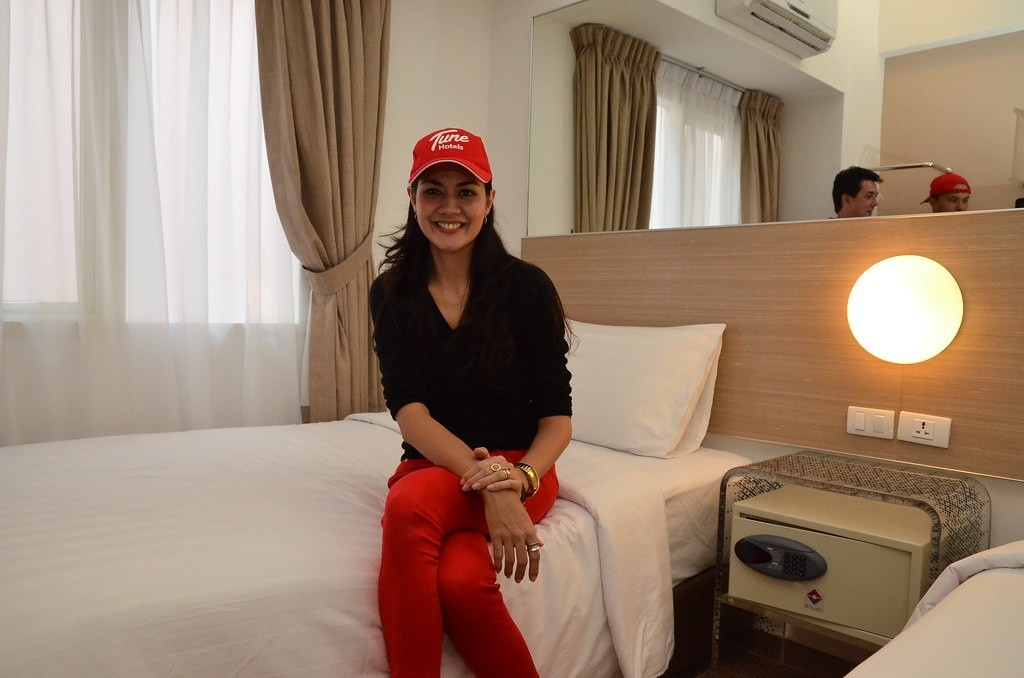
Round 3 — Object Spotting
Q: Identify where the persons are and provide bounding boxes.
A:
[920,174,971,213]
[368,128,572,678]
[828,166,883,219]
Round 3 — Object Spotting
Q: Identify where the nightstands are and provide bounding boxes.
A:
[706,447,992,678]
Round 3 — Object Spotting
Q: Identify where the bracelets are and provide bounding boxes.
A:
[514,463,539,498]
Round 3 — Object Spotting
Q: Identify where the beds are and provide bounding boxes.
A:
[0,415,751,678]
[841,542,1024,678]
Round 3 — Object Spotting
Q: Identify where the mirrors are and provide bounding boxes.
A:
[528,0,1023,237]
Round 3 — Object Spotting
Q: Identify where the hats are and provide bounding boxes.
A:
[918,172,972,205]
[407,127,494,186]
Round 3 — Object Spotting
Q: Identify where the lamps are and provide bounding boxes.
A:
[845,254,964,365]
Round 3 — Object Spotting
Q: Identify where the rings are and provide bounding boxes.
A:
[526,542,539,552]
[491,464,501,471]
[504,469,510,478]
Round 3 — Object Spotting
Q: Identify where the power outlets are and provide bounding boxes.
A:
[897,410,952,450]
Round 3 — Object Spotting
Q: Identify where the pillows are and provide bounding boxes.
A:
[559,314,729,461]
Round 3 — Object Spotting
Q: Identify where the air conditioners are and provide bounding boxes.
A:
[714,0,840,61]
